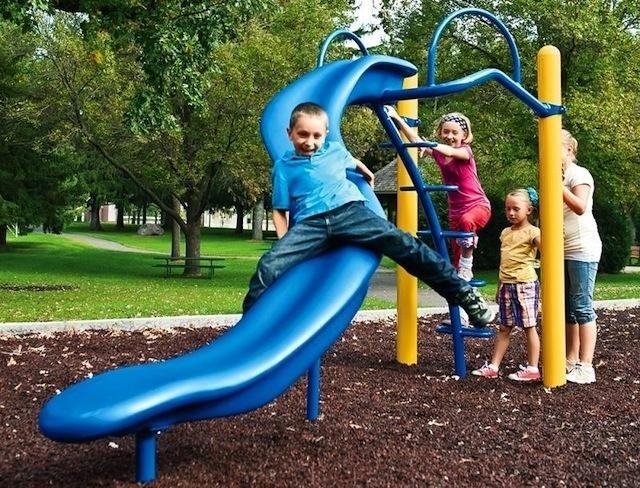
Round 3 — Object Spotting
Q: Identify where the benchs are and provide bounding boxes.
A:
[152,265,225,275]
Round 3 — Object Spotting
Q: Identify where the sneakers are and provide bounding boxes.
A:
[457,267,473,283]
[471,361,499,379]
[457,287,492,326]
[507,364,541,382]
[566,359,596,385]
[442,315,470,327]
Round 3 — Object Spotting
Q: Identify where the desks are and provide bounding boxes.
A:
[264,237,279,249]
[153,256,225,279]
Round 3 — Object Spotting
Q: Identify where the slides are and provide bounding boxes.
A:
[39,55,419,444]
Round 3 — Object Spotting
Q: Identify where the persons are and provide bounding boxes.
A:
[471,187,542,381]
[384,105,492,282]
[243,103,491,329]
[561,129,603,384]
